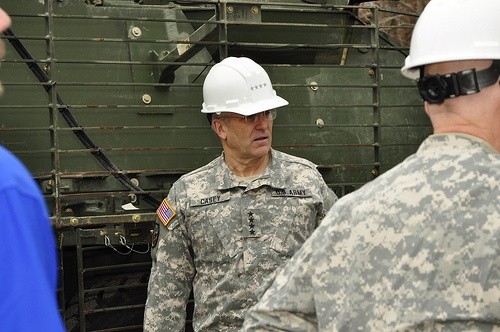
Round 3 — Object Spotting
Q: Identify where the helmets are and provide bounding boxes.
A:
[200,56,290,116]
[401,0,500,80]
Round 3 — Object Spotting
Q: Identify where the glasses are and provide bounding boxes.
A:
[212,107,277,125]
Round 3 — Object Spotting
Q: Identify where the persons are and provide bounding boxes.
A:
[0,6,69,332]
[240,0,500,332]
[143,56,338,332]
